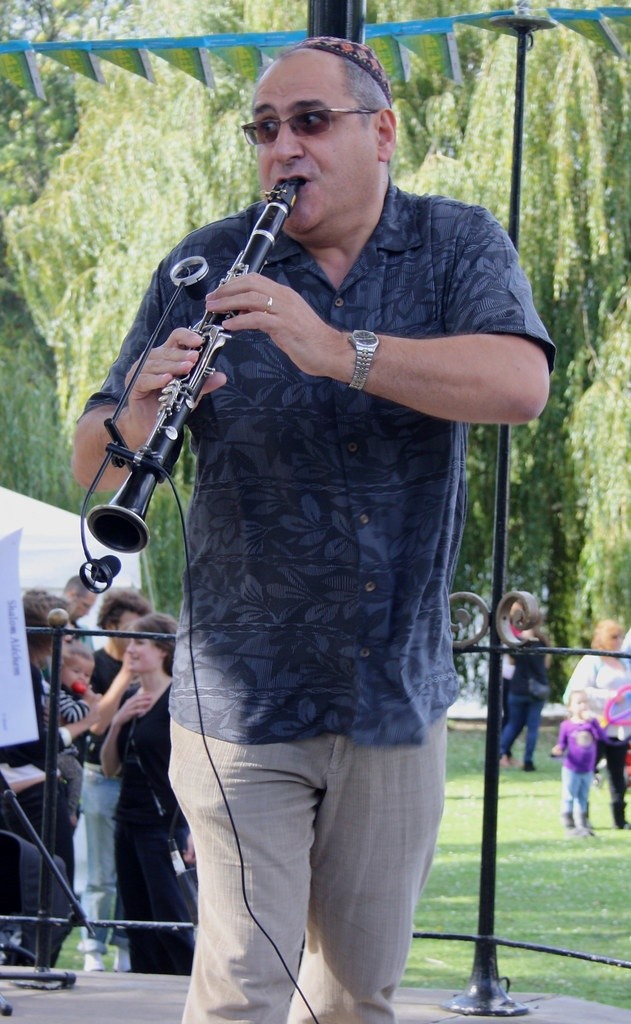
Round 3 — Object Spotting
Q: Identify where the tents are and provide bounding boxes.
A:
[0,484,142,660]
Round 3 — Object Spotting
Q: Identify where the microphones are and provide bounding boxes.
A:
[80,555,121,594]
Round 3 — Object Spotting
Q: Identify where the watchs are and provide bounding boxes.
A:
[346,329,379,392]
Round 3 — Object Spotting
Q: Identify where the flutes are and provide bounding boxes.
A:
[85,174,304,555]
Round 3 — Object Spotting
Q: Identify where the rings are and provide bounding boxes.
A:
[263,297,273,312]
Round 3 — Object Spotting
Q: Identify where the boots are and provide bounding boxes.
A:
[608,803,631,829]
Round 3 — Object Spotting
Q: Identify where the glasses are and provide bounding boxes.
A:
[240,108,380,147]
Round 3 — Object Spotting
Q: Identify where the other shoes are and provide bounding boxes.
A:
[112,947,130,971]
[524,761,534,771]
[563,814,593,829]
[85,952,105,971]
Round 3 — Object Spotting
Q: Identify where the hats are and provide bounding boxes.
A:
[294,36,394,109]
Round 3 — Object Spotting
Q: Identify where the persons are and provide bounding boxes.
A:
[67,37,555,1024]
[0,600,631,976]
